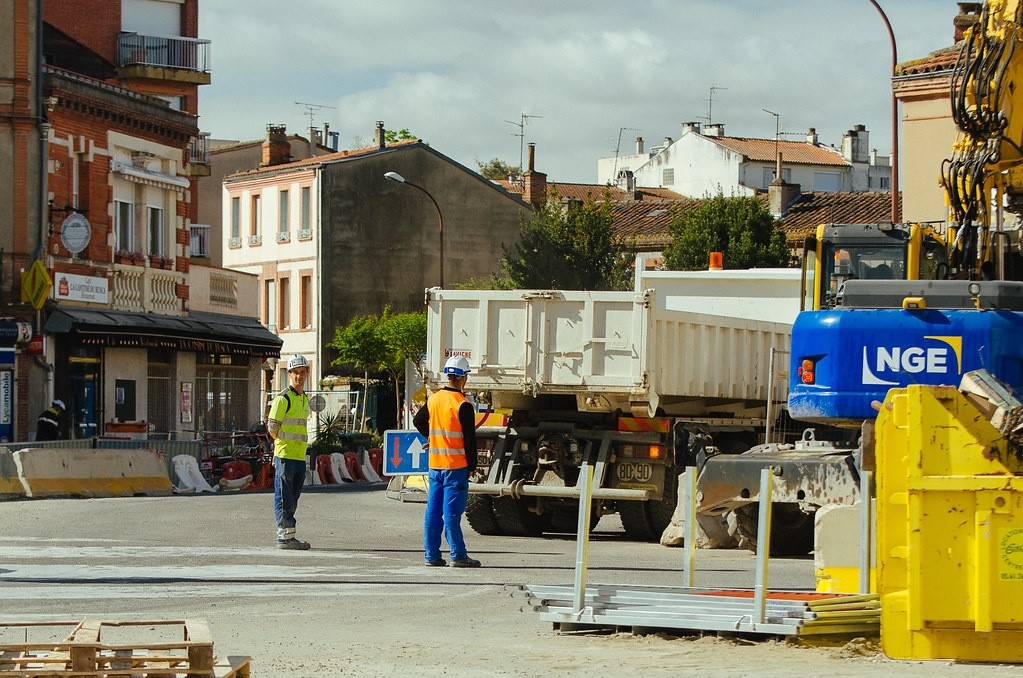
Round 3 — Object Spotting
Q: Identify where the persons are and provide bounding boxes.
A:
[35,400,65,442]
[413,356,481,567]
[268,354,311,551]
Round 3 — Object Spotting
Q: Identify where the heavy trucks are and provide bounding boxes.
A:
[422,251,897,663]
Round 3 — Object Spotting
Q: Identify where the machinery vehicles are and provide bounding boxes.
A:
[671,1,1023,664]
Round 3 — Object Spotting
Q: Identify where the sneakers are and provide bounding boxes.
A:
[426,558,446,566]
[450,555,481,567]
[277,538,311,550]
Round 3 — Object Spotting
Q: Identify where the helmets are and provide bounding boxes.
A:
[52,399,66,410]
[287,354,309,373]
[444,355,471,376]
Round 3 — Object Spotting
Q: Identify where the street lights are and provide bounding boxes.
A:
[385,171,444,289]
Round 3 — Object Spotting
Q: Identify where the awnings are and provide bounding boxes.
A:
[47,305,283,359]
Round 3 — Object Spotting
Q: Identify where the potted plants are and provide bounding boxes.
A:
[209,411,375,483]
